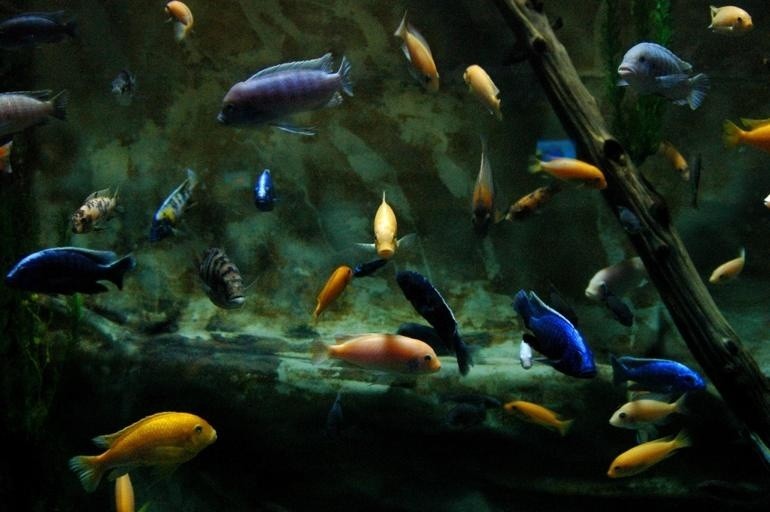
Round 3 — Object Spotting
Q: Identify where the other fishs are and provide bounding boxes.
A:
[308,0,770,477]
